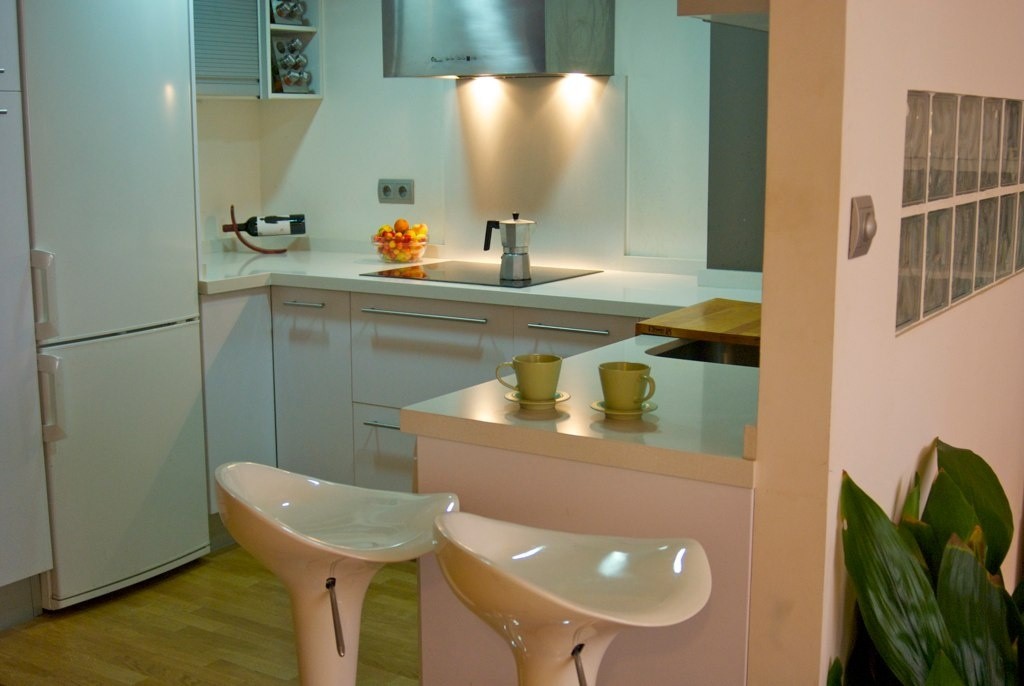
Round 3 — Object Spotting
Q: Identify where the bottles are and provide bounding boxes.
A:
[271,1,303,24]
[274,38,311,91]
[222,214,308,237]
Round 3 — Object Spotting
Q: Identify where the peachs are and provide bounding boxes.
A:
[377,266,428,279]
[374,223,429,262]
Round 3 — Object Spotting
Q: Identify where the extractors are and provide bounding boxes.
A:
[378,1,616,80]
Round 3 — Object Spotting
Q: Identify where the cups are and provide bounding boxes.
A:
[495,353,562,400]
[597,361,654,411]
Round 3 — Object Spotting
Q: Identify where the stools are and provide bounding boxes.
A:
[433,507,715,685]
[216,455,459,686]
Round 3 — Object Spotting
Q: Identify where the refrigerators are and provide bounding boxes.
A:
[14,2,215,611]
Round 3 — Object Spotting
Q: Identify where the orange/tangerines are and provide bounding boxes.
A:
[394,219,410,233]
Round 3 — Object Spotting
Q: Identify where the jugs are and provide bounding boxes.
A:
[483,214,538,254]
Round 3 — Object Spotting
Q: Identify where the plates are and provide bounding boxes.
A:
[591,401,658,419]
[504,389,571,409]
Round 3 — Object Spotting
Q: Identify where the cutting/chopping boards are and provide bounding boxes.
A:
[634,296,761,352]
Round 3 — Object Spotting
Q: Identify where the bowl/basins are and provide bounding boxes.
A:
[371,240,428,262]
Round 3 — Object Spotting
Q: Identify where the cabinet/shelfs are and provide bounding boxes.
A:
[266,275,643,494]
